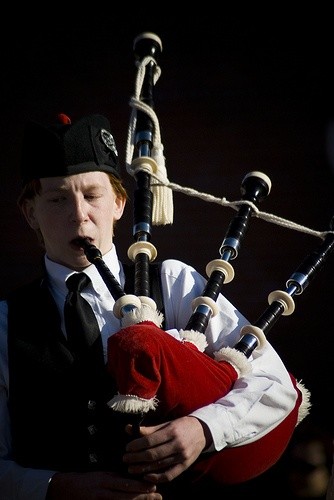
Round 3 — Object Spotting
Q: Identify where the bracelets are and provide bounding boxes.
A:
[42,470,64,499]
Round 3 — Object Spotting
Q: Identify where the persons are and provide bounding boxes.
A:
[0,113,306,499]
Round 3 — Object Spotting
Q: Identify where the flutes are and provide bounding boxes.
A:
[77,32,333,500]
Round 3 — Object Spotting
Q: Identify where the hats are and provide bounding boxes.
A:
[17,110,121,187]
